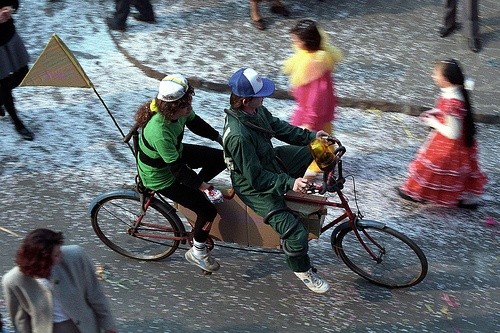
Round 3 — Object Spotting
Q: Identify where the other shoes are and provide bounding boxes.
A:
[133,14,151,21]
[398,191,421,202]
[441,24,453,37]
[253,18,264,29]
[271,6,290,17]
[0,107,6,116]
[15,125,35,140]
[471,37,481,53]
[457,200,479,210]
[106,17,125,30]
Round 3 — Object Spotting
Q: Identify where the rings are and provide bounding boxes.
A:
[302,179,304,182]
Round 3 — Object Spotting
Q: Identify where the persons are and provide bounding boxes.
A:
[438,0,483,53]
[0,0,37,142]
[0,228,124,333]
[247,0,293,30]
[222,67,330,294]
[396,56,500,211]
[104,0,158,33]
[280,19,342,135]
[135,73,227,272]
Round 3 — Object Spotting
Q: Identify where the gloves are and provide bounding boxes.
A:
[204,185,224,205]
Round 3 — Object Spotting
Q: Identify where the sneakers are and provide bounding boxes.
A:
[294,267,330,294]
[185,245,220,272]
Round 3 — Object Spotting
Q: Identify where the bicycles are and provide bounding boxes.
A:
[87,122,428,291]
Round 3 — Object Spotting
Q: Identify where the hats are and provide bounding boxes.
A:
[156,72,188,102]
[228,67,276,97]
